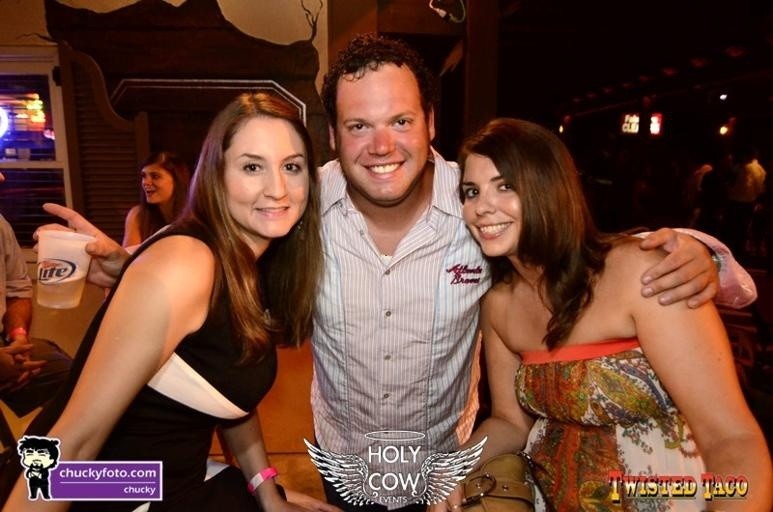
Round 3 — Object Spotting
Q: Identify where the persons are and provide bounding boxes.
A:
[0,173,74,511]
[119,148,190,250]
[578,141,772,266]
[423,114,772,512]
[31,32,762,512]
[0,90,349,510]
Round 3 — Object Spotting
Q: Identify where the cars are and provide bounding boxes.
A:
[32,229,95,309]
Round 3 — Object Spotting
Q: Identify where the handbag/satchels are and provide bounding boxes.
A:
[246,465,277,492]
